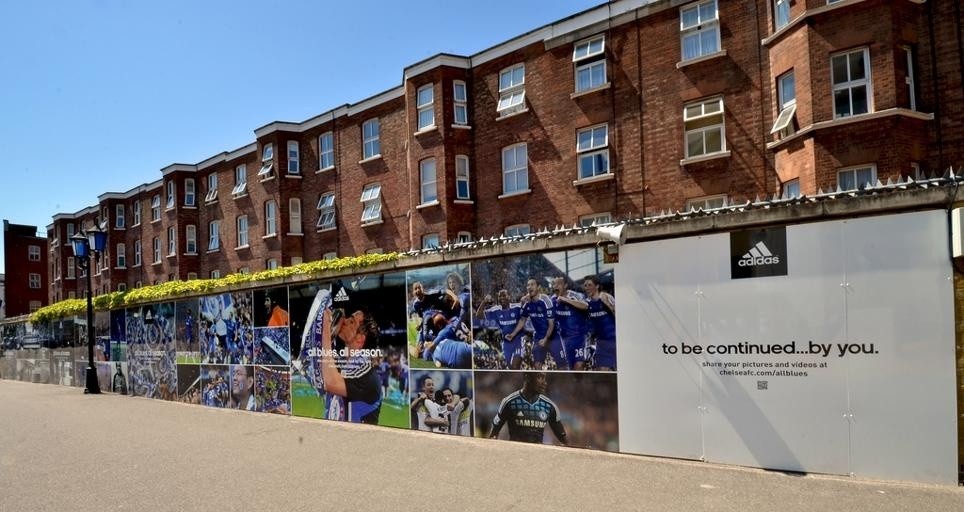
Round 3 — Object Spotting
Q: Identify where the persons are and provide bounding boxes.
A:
[113,273,616,447]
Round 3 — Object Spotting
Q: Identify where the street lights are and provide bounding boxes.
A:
[69,225,108,393]
